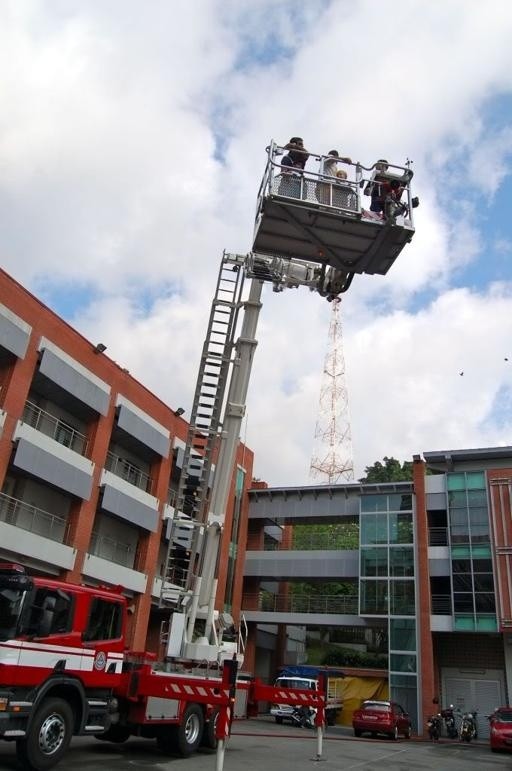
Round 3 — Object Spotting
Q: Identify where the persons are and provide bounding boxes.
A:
[313,147,354,205]
[363,158,407,211]
[336,166,350,211]
[278,135,310,204]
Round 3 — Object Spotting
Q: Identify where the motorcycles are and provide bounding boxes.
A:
[460,709,478,741]
[427,713,442,740]
[440,704,458,739]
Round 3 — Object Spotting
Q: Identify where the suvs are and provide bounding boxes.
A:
[353,699,413,740]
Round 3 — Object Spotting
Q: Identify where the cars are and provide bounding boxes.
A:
[485,706,511,753]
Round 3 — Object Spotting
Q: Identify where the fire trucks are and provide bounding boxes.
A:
[0,137,419,770]
[269,665,345,726]
